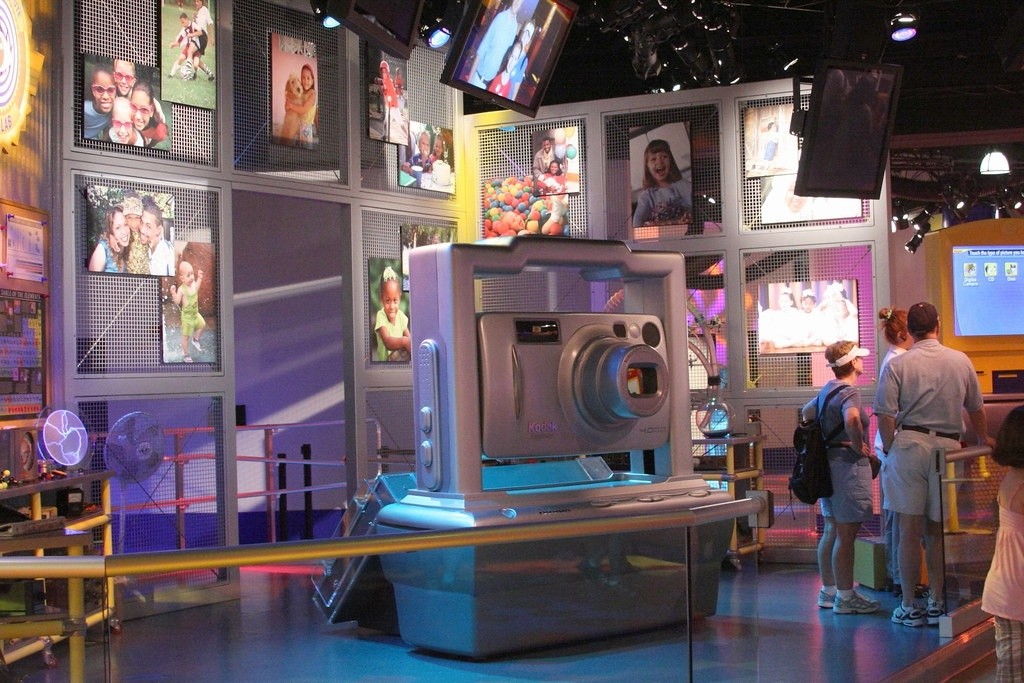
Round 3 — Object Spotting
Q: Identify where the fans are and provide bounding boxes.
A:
[105,411,170,604]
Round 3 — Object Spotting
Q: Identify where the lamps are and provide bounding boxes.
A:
[418,6,452,49]
[979,145,1011,174]
[772,42,797,71]
[891,196,1022,254]
[889,2,919,44]
[309,0,343,28]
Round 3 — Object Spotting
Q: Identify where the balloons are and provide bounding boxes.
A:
[555,144,577,164]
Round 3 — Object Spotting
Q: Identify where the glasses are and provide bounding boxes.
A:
[129,102,154,114]
[112,71,134,83]
[90,83,118,95]
[111,118,134,128]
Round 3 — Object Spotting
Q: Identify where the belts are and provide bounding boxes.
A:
[897,425,961,442]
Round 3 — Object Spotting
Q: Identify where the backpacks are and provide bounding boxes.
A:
[789,384,851,505]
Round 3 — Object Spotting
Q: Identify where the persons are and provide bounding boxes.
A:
[168,0,215,81]
[813,70,887,193]
[89,193,174,276]
[374,267,412,361]
[432,234,440,244]
[533,138,568,195]
[798,341,881,614]
[980,405,1024,683]
[761,173,817,223]
[169,261,206,363]
[758,283,859,347]
[631,139,692,227]
[501,196,568,236]
[84,58,167,147]
[875,308,932,598]
[469,0,535,101]
[285,63,317,149]
[873,303,994,628]
[376,60,454,176]
[761,121,781,161]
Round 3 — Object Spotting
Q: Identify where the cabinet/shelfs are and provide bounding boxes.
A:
[0,465,121,670]
[691,436,768,571]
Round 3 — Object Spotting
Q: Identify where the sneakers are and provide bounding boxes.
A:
[925,597,944,626]
[817,588,839,607]
[833,589,880,613]
[892,602,925,627]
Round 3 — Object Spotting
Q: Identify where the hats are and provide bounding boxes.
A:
[524,22,535,38]
[122,197,143,218]
[826,344,870,368]
[906,302,940,333]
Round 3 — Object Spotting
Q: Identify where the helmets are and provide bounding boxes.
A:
[380,61,391,77]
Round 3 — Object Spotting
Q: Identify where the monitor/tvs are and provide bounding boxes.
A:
[794,58,904,200]
[953,245,1024,335]
[326,0,425,60]
[438,0,580,118]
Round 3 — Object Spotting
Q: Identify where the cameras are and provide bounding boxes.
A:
[473,311,673,460]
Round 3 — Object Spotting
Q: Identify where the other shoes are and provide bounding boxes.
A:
[182,352,194,364]
[191,338,202,352]
[168,63,215,83]
[893,583,931,598]
[885,579,892,591]
